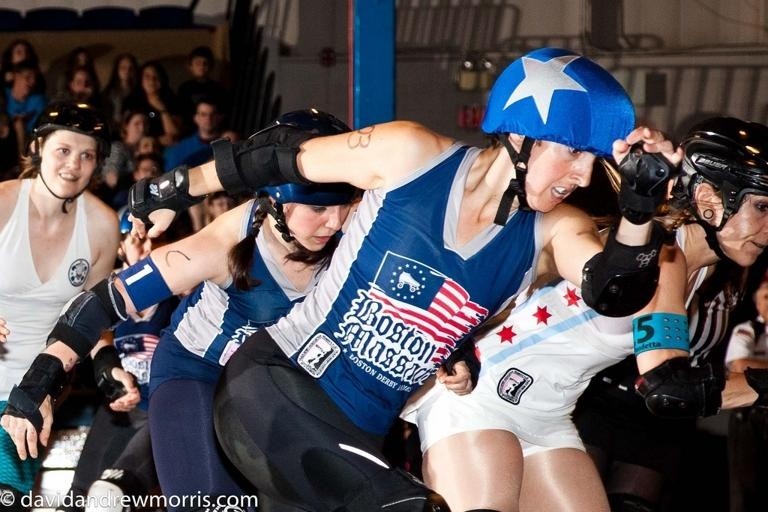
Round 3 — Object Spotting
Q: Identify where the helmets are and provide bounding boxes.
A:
[256,108,360,206]
[481,48,635,156]
[679,117,767,216]
[32,99,111,160]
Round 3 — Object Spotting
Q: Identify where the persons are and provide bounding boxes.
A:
[0,36,768,511]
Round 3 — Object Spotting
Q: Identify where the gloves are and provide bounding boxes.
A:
[617,140,677,225]
[744,366,767,406]
[92,345,140,406]
[128,166,205,224]
[444,335,482,390]
[1,354,68,433]
